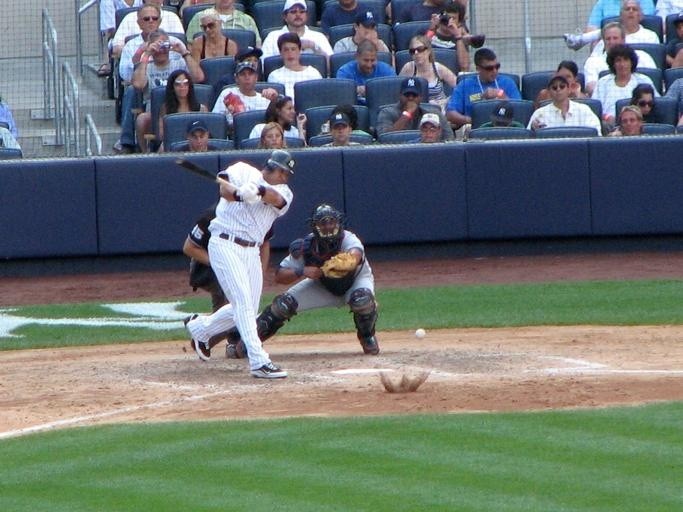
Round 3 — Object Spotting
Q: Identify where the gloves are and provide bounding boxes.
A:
[239,183,261,204]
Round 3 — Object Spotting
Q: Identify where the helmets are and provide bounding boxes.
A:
[266,150,295,175]
[310,205,344,252]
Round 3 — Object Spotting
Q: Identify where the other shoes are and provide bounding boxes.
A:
[116,145,135,154]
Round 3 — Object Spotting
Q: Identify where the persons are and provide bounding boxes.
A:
[183,150,295,378]
[528,0,683,135]
[234,203,379,359]
[184,213,276,343]
[100,1,520,151]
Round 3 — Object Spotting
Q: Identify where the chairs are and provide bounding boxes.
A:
[105,0,683,154]
[0,148,22,161]
[0,119,9,130]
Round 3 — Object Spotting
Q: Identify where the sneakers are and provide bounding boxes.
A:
[183,314,380,379]
[563,35,585,50]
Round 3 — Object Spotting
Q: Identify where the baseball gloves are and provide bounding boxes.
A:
[322,254,356,279]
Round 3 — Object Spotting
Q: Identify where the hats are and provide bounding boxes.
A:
[190,121,207,133]
[674,13,683,23]
[401,77,422,94]
[419,113,439,128]
[548,74,570,87]
[330,112,350,127]
[492,102,513,123]
[235,62,257,74]
[356,7,378,26]
[235,46,263,60]
[282,0,308,11]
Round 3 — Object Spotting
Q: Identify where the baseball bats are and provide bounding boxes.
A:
[177,159,243,191]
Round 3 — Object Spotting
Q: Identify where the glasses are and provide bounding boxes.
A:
[409,46,428,55]
[276,94,284,106]
[200,22,218,31]
[421,126,438,132]
[475,63,500,71]
[550,84,566,90]
[174,78,189,86]
[638,99,654,106]
[402,92,419,97]
[140,15,160,21]
[287,8,306,14]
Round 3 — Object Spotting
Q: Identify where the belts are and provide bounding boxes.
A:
[221,233,261,247]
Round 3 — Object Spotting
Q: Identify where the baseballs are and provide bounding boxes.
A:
[416,329,425,339]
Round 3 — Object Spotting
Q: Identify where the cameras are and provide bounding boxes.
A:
[439,14,450,24]
[159,40,170,49]
